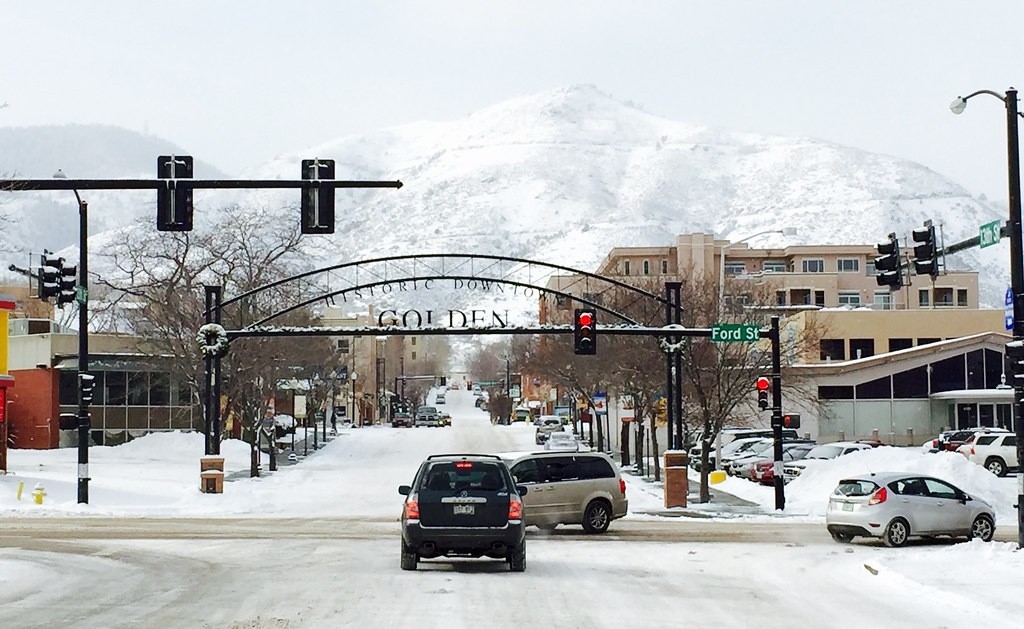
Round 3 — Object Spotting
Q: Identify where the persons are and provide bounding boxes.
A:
[331,413,339,429]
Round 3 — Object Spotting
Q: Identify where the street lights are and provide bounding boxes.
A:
[52,169,90,504]
[950,87,1024,551]
[715,226,797,471]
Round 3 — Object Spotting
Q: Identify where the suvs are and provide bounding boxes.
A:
[399,453,528,571]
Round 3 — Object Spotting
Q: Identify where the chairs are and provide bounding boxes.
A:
[888,482,914,495]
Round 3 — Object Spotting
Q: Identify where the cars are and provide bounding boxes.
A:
[544,432,579,452]
[685,428,873,488]
[473,387,568,426]
[929,428,1019,477]
[391,394,452,428]
[826,472,997,547]
[535,425,565,445]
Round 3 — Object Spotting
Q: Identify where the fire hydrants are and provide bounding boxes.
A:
[31,483,47,504]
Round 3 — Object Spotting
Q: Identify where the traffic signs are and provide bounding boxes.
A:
[979,220,1000,248]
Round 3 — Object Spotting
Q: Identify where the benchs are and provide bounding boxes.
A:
[429,473,502,490]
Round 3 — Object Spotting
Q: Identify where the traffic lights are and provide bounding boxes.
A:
[757,377,769,407]
[875,232,903,291]
[37,249,77,310]
[574,308,596,355]
[784,414,800,428]
[912,220,939,279]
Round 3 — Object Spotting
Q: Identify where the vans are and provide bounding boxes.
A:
[493,449,628,535]
